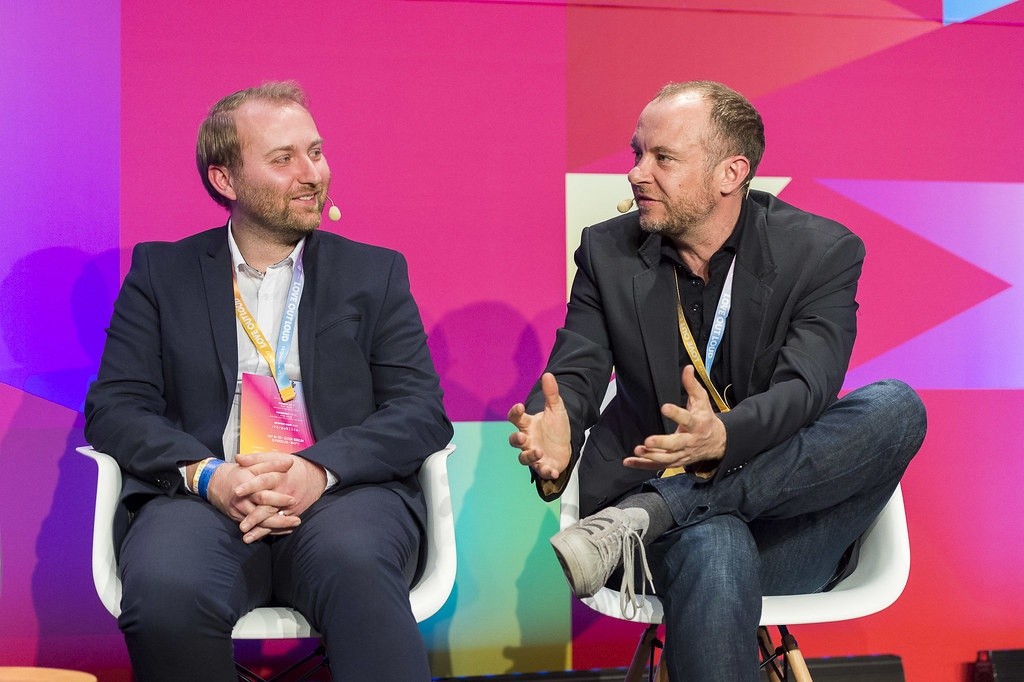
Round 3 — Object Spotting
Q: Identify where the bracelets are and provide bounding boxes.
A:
[191,457,226,503]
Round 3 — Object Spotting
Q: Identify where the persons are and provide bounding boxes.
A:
[506,78,929,682]
[83,87,455,682]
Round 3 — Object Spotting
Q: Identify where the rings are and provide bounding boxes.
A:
[277,510,286,517]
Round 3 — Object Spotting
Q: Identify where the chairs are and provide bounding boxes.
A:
[558,379,910,682]
[77,442,460,682]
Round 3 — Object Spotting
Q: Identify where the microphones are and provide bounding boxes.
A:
[326,196,341,221]
[617,198,635,213]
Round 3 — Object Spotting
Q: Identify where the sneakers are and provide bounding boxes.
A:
[550,507,649,598]
